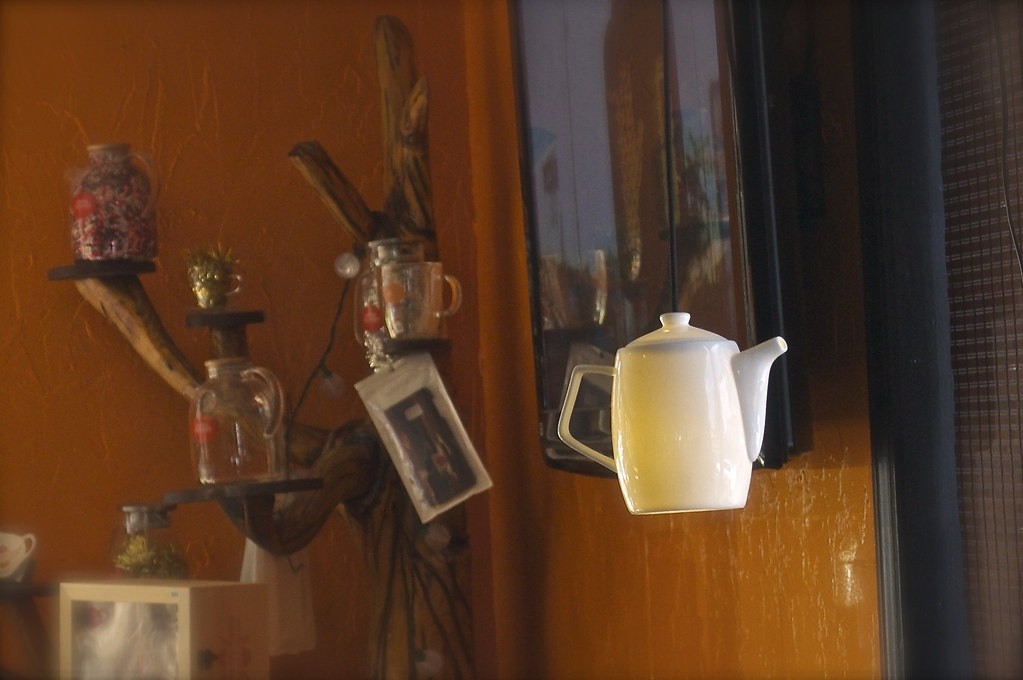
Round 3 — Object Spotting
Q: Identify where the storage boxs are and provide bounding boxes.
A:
[59,578,273,680]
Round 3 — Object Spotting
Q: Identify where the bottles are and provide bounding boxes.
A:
[112,501,189,580]
[70,142,161,264]
[352,238,426,352]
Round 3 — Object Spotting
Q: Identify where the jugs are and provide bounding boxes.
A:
[182,357,289,486]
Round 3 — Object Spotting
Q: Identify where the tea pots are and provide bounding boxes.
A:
[556,312,789,516]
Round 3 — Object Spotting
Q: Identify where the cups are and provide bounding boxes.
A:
[380,260,462,340]
